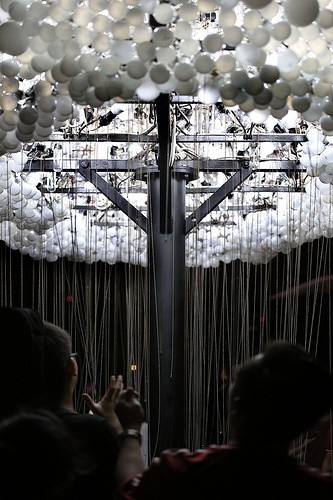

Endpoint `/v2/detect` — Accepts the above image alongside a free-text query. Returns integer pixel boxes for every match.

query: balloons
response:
[0,0,333,269]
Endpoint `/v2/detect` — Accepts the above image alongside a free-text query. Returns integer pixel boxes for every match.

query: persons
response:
[114,341,333,500]
[0,306,124,500]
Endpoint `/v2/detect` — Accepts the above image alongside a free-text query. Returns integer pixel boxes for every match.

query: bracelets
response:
[120,429,142,446]
